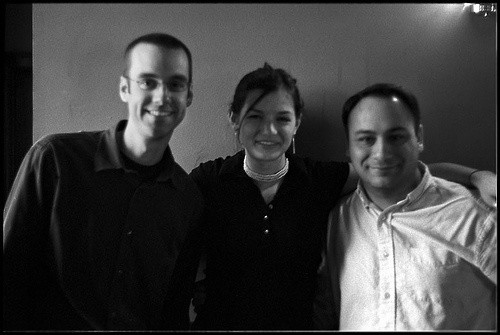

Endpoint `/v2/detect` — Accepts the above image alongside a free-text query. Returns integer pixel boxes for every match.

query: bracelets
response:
[469,169,482,187]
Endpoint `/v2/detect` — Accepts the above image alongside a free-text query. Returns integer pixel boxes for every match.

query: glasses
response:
[127,76,189,92]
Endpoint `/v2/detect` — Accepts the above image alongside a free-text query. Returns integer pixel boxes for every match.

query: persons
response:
[188,62,497,332]
[2,33,203,332]
[314,83,497,332]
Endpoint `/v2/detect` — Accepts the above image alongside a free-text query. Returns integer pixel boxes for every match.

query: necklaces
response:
[242,155,289,181]
